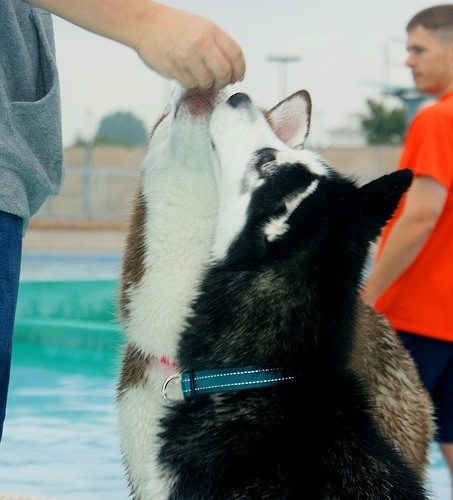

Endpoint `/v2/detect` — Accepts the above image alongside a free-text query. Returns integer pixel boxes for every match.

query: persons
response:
[358,4,453,478]
[0,0,244,445]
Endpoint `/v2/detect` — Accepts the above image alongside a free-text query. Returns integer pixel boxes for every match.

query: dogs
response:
[115,79,440,500]
[152,92,430,499]
